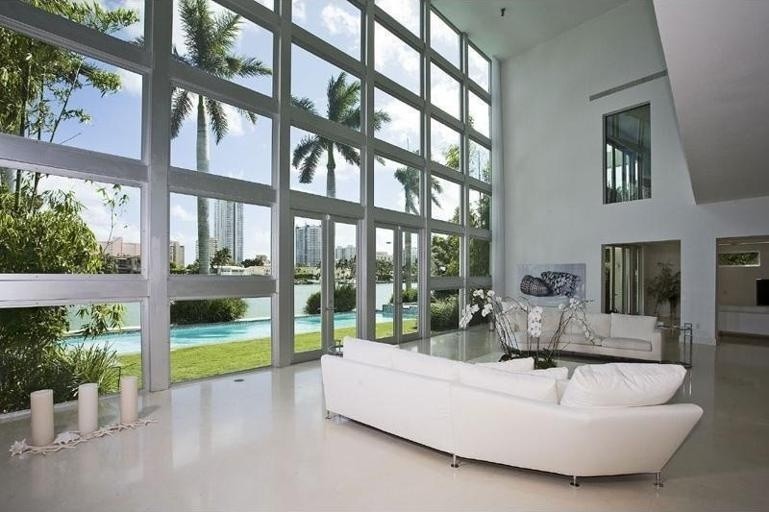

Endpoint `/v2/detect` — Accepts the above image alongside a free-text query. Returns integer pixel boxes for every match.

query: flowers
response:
[457,289,599,339]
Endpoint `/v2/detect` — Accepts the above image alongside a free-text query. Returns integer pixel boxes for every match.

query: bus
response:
[221,266,245,275]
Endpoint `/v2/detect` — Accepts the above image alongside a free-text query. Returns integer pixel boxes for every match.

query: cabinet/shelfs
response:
[717,306,769,340]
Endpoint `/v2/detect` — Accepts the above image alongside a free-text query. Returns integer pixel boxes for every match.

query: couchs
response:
[319,305,703,489]
